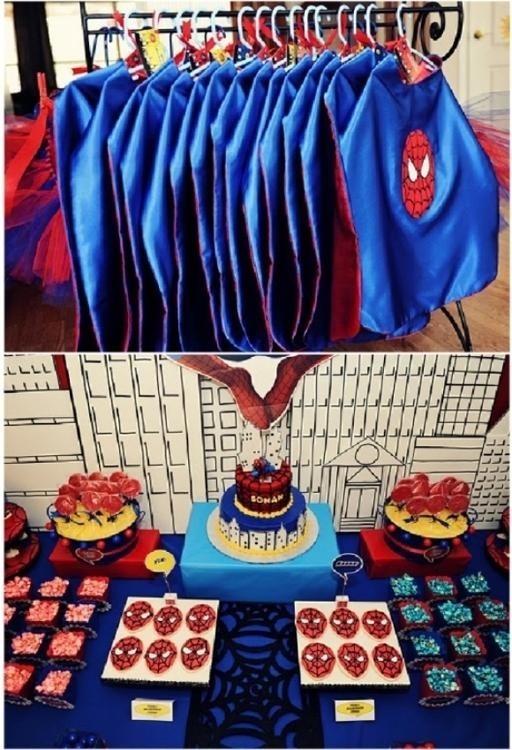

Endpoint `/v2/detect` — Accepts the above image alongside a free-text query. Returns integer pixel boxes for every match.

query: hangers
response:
[113,2,440,66]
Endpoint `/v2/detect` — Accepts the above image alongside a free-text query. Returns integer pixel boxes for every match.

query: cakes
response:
[5,502,31,566]
[384,473,471,539]
[217,455,308,552]
[47,471,142,542]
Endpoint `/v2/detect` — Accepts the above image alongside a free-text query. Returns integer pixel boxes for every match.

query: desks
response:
[6,521,509,747]
